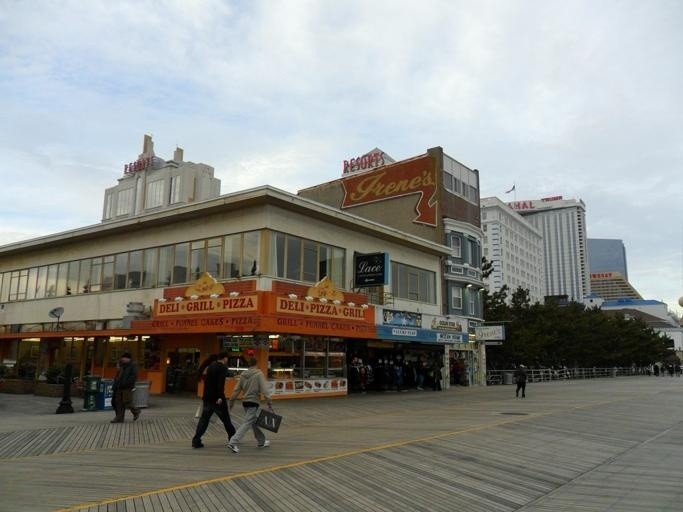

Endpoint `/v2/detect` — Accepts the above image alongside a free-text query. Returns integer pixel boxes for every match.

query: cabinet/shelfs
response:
[220,335,347,380]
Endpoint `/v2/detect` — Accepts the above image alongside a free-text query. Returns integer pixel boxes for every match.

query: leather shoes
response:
[133,415,138,420]
[191,443,203,448]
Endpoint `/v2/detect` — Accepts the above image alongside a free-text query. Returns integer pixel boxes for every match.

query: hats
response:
[520,365,524,368]
[246,356,256,366]
[218,351,230,357]
[121,353,131,359]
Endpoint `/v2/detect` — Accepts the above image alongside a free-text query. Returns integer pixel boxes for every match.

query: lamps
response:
[158,291,239,304]
[479,287,484,290]
[289,293,368,311]
[466,283,472,287]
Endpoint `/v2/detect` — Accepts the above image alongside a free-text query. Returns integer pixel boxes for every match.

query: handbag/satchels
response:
[512,375,517,384]
[255,408,282,433]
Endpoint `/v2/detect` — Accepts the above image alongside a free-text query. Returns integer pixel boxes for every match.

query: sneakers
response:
[227,444,239,453]
[258,440,270,448]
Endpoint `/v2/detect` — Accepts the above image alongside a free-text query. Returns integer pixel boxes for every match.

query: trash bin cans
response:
[504,373,514,385]
[82,375,151,411]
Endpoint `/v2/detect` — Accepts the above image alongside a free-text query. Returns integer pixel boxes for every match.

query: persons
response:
[110,353,140,423]
[192,351,236,448]
[225,356,272,452]
[395,358,463,392]
[195,353,217,418]
[513,364,527,397]
[110,359,122,416]
[645,361,682,377]
